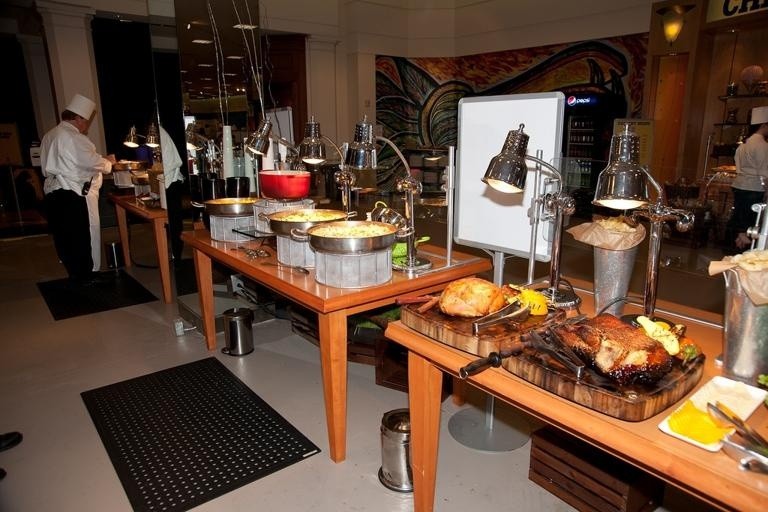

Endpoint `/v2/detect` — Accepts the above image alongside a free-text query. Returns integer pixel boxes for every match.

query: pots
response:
[257,209,416,254]
[108,162,150,186]
[190,198,263,216]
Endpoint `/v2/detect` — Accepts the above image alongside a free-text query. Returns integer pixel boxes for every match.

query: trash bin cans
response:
[664,179,701,209]
[104,240,125,269]
[221,307,255,356]
[320,160,355,203]
[378,408,413,493]
[668,200,711,247]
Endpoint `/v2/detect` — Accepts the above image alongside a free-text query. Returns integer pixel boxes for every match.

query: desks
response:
[113,190,184,300]
[383,275,768,512]
[179,224,490,465]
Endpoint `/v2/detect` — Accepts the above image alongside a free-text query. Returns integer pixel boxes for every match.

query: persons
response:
[720,105,768,256]
[0,430,23,482]
[39,92,118,289]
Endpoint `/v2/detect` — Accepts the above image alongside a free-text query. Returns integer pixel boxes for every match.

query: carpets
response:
[34,268,161,325]
[80,354,324,512]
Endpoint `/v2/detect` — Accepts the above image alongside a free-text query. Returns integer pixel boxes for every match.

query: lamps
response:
[484,121,581,311]
[183,120,244,198]
[594,118,695,318]
[123,122,167,211]
[342,114,432,271]
[247,116,357,222]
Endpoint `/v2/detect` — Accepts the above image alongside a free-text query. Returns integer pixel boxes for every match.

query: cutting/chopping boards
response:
[401,284,705,423]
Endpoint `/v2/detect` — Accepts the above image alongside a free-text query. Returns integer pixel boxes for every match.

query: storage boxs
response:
[284,303,452,401]
[523,427,662,512]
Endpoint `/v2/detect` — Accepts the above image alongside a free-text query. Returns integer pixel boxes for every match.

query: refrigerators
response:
[562,90,628,198]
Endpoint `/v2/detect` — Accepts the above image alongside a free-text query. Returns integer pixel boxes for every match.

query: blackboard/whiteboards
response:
[454,91,565,263]
[0,123,24,167]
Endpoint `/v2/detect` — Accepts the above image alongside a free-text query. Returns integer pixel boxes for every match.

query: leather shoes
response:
[0,430,23,452]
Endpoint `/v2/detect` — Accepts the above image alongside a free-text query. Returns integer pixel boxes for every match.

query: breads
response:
[555,312,673,388]
[438,277,506,319]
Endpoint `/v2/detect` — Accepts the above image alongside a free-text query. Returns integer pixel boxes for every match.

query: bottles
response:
[568,116,597,187]
[736,127,746,144]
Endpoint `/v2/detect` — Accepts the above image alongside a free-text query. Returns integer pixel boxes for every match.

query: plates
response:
[658,376,767,452]
[712,166,737,172]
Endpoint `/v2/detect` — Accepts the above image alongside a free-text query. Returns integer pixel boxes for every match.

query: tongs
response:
[706,400,767,455]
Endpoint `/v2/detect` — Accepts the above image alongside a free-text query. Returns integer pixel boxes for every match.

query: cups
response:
[592,246,637,316]
[726,81,767,125]
[190,172,249,198]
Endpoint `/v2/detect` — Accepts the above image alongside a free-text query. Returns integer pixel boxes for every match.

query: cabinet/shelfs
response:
[708,91,768,158]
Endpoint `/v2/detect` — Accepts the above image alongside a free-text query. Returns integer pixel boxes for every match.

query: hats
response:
[65,94,97,120]
[750,106,768,125]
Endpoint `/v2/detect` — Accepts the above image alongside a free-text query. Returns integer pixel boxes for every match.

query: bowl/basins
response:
[372,201,430,258]
[141,197,161,209]
[258,169,310,199]
[666,184,700,199]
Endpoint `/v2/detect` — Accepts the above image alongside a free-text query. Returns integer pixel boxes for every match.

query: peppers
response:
[676,337,703,366]
